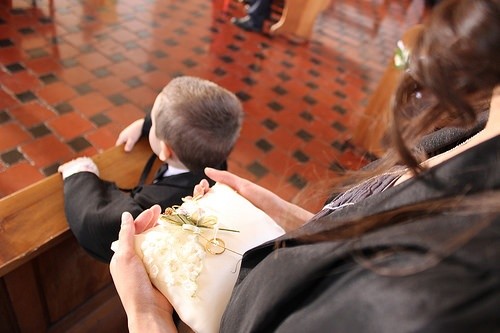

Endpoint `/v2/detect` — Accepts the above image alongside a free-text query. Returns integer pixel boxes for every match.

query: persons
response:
[232,0,272,33]
[109,0,500,333]
[58,75,243,261]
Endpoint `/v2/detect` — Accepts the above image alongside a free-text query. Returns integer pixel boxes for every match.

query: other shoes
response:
[231,14,263,31]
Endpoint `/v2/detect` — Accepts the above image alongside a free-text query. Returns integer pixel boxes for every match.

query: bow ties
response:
[152,163,168,180]
